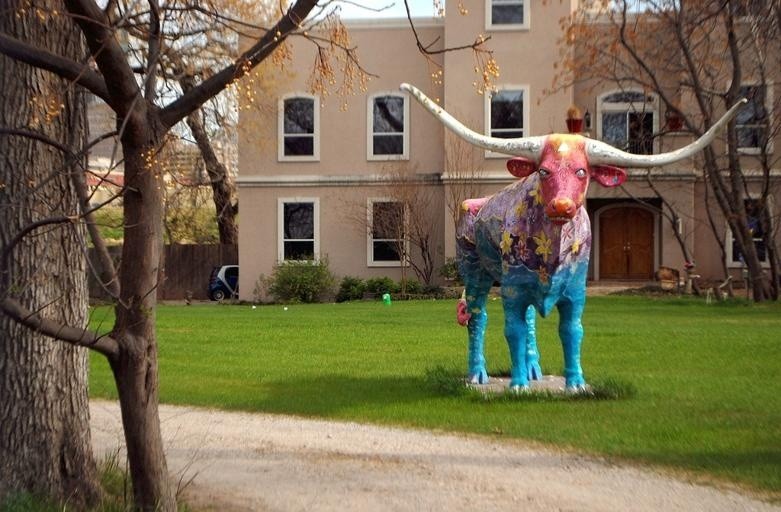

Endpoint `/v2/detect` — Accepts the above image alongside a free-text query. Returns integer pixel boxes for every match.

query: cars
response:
[208,264,238,302]
